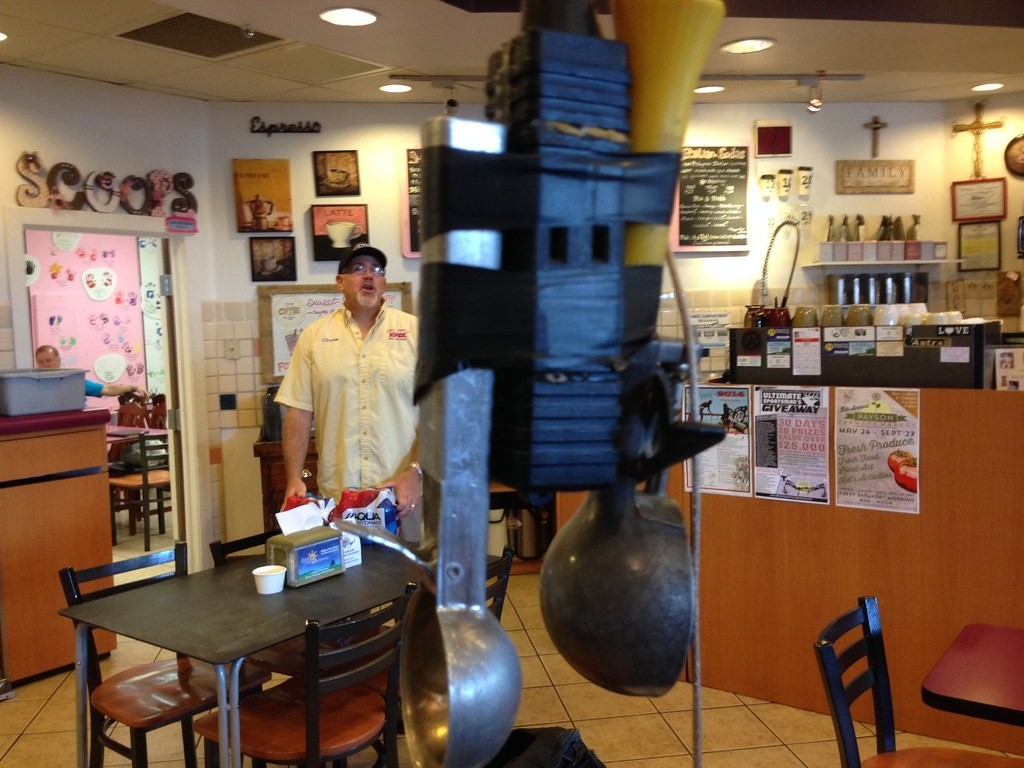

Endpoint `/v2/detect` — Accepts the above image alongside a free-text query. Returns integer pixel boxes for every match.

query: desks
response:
[922,624,1024,729]
[106,422,168,444]
[57,554,436,768]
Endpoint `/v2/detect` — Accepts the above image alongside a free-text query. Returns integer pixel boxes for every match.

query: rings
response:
[411,504,416,508]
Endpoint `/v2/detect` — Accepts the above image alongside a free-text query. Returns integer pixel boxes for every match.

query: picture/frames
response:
[248,236,298,282]
[952,176,1007,221]
[310,204,370,262]
[311,150,361,197]
[959,222,1002,270]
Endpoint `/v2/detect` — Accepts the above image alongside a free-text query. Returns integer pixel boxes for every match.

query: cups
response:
[790,302,963,325]
[258,240,277,271]
[253,564,287,594]
[326,222,363,248]
[328,167,348,183]
[762,307,791,328]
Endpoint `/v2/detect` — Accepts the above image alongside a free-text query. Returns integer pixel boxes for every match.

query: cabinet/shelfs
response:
[0,409,117,689]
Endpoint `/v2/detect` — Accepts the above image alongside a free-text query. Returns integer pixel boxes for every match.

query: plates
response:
[324,178,350,188]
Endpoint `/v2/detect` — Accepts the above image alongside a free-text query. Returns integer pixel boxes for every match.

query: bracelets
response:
[132,383,138,391]
[408,462,423,479]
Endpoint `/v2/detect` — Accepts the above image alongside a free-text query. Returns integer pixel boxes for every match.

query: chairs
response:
[108,391,173,551]
[814,595,1024,768]
[191,582,417,768]
[209,528,333,677]
[367,545,515,736]
[59,540,272,768]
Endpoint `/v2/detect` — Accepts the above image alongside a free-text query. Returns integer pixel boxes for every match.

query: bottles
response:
[744,304,766,327]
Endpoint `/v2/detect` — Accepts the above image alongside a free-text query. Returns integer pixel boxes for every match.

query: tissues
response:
[265,502,345,588]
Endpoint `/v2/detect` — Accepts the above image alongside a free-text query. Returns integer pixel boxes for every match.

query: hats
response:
[338,243,388,276]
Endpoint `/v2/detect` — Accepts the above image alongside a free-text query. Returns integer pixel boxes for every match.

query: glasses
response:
[342,264,386,277]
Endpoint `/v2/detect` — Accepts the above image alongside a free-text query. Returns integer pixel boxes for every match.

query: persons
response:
[36,345,151,409]
[722,404,733,431]
[700,400,714,421]
[273,242,424,735]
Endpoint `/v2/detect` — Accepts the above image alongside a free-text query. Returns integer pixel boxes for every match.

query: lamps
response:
[806,85,824,112]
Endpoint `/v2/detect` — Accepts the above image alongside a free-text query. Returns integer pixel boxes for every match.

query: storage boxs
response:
[820,240,948,262]
[0,368,92,417]
